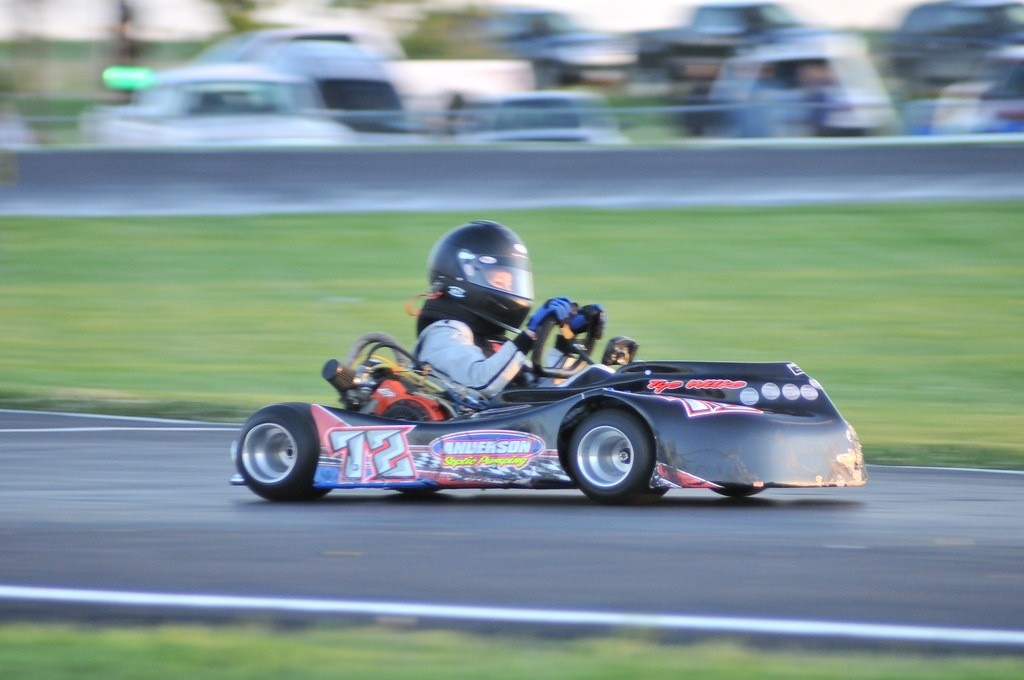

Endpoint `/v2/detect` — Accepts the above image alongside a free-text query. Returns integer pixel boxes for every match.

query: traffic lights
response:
[98,64,150,90]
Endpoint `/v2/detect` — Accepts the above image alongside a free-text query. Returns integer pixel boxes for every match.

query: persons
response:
[414,221,605,406]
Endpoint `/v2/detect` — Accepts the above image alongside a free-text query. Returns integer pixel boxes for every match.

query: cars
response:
[79,0,1022,145]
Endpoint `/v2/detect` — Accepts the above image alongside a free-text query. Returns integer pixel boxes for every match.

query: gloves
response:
[568,304,605,336]
[526,297,576,333]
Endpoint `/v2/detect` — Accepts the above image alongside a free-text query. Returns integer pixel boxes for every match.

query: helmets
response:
[428,220,535,335]
